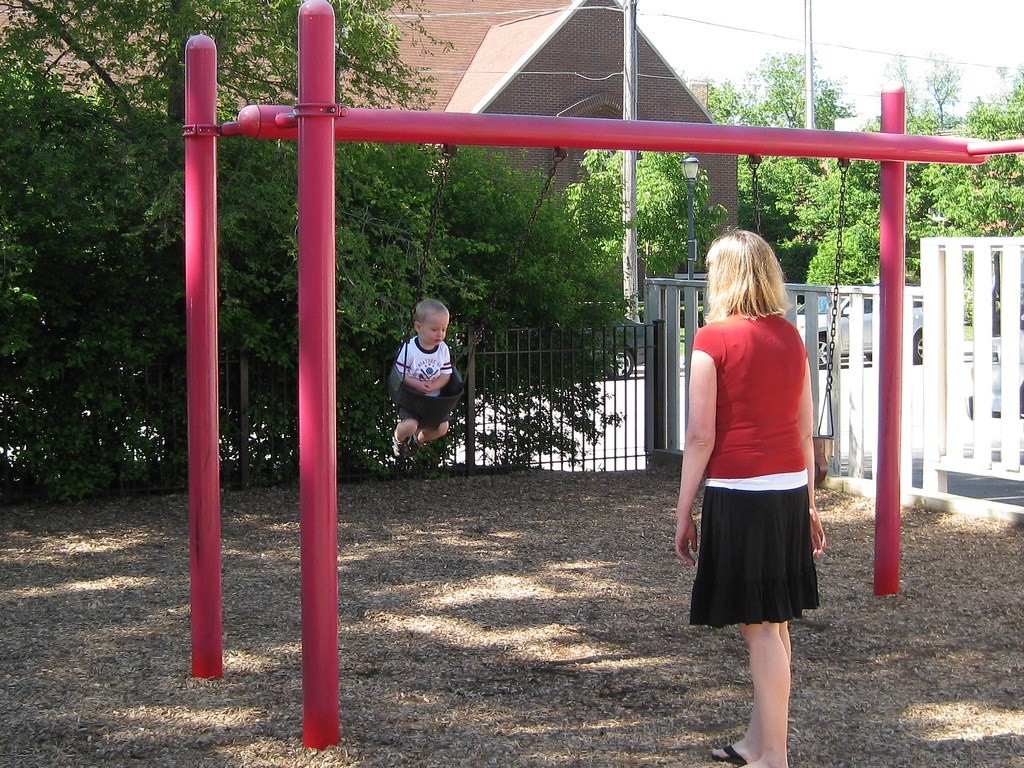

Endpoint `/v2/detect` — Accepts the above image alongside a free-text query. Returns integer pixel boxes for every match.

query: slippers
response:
[711,745,748,765]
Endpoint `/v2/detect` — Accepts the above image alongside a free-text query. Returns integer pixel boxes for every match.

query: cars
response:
[796,284,922,365]
[574,312,646,382]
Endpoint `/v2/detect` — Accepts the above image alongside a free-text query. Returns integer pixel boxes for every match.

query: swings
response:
[751,163,848,487]
[388,150,566,429]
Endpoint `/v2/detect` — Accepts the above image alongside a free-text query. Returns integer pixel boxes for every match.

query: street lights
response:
[678,154,701,280]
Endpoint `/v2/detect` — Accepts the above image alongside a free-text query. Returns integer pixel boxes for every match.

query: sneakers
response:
[392,431,407,459]
[407,427,425,456]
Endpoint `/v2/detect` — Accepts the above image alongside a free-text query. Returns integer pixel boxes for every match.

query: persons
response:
[392,299,452,458]
[675,230,827,768]
